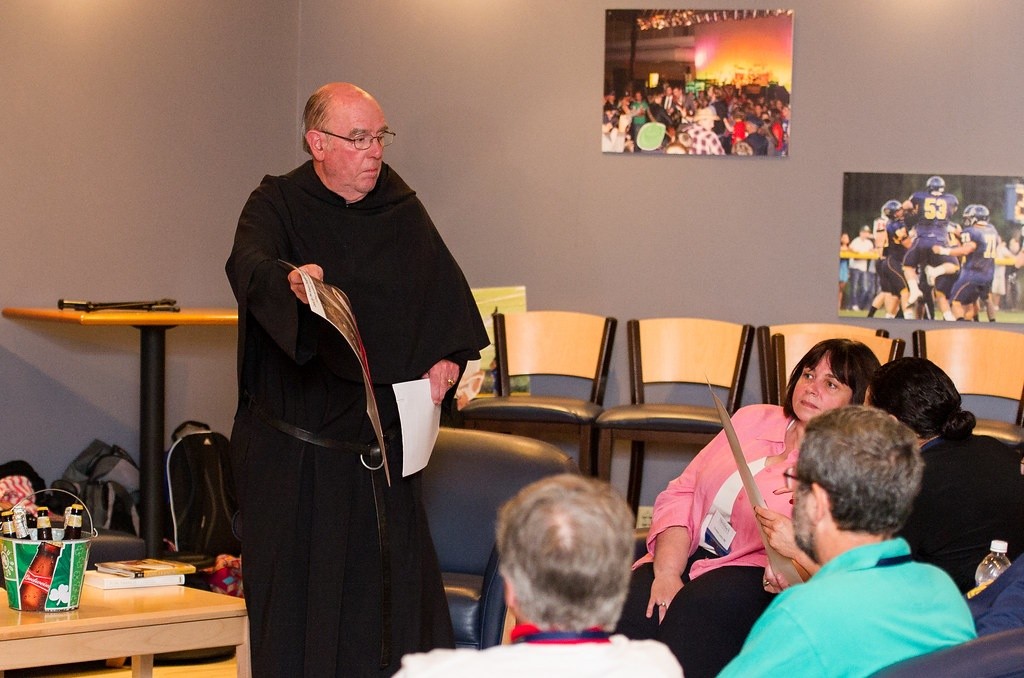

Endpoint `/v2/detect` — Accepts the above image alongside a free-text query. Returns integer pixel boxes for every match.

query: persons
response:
[601,80,791,157]
[839,176,1024,323]
[391,338,1024,678]
[225,83,491,677]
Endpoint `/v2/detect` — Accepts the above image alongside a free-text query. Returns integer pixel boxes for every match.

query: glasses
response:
[783,467,842,496]
[317,131,396,150]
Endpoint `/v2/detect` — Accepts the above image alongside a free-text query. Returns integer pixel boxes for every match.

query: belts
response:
[239,398,401,669]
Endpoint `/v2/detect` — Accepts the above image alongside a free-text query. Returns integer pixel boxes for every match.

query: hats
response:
[859,224,871,232]
[741,112,763,127]
[636,122,666,150]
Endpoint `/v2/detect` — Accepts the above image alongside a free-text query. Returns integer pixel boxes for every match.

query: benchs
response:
[0,521,147,667]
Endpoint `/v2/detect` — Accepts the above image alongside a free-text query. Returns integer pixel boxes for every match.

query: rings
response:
[449,379,455,386]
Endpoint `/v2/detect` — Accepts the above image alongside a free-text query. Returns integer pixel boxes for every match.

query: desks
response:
[0,570,253,678]
[0,306,242,668]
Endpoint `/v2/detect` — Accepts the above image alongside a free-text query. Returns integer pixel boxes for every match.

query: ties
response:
[666,99,670,110]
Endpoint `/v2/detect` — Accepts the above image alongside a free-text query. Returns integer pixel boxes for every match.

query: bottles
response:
[974,539,1012,588]
[19,541,65,611]
[1,502,84,540]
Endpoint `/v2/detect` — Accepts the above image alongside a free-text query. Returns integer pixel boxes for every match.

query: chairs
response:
[415,313,1024,678]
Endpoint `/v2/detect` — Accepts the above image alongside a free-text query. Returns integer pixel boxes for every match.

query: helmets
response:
[944,194,959,206]
[926,176,945,189]
[883,200,900,216]
[963,204,991,218]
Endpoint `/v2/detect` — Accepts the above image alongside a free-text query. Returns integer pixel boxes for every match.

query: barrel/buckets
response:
[0,489,98,612]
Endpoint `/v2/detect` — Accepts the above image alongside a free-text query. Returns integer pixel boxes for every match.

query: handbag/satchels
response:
[51,440,141,515]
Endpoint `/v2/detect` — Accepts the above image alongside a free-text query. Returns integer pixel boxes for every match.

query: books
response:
[84,558,197,589]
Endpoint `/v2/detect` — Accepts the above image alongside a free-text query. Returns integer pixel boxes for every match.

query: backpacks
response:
[76,480,140,538]
[161,419,238,560]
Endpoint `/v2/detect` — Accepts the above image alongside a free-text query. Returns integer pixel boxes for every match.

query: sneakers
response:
[905,291,923,308]
[924,265,935,288]
